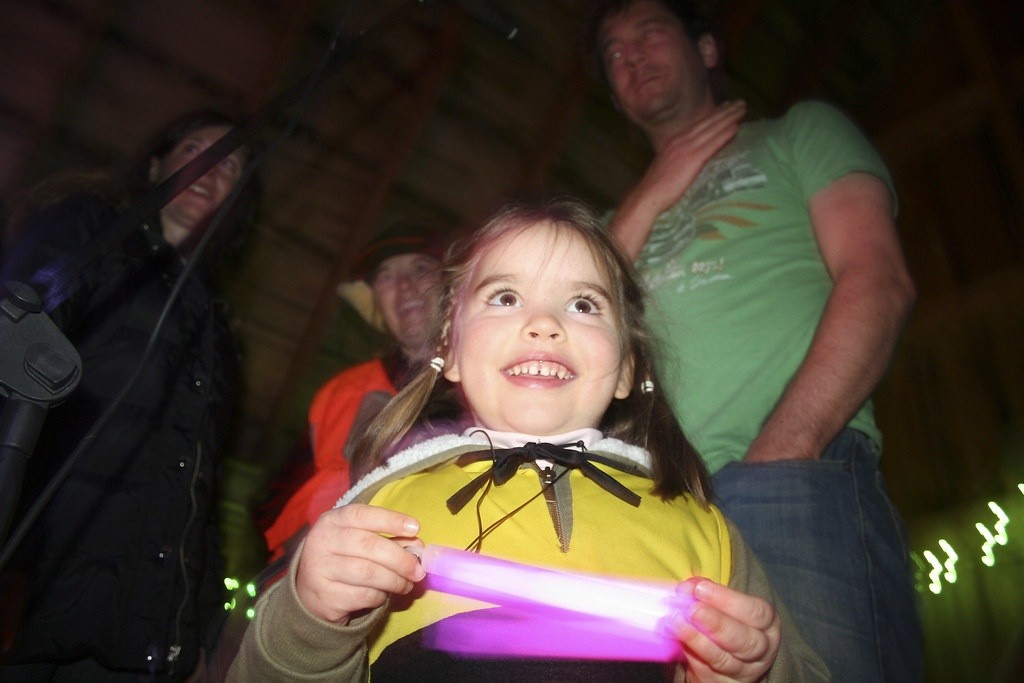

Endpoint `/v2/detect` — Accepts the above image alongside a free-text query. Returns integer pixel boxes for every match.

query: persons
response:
[253,224,475,596]
[0,109,266,683]
[592,0,935,682]
[217,199,833,683]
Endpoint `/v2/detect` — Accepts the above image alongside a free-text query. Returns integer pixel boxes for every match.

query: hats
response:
[336,221,453,336]
